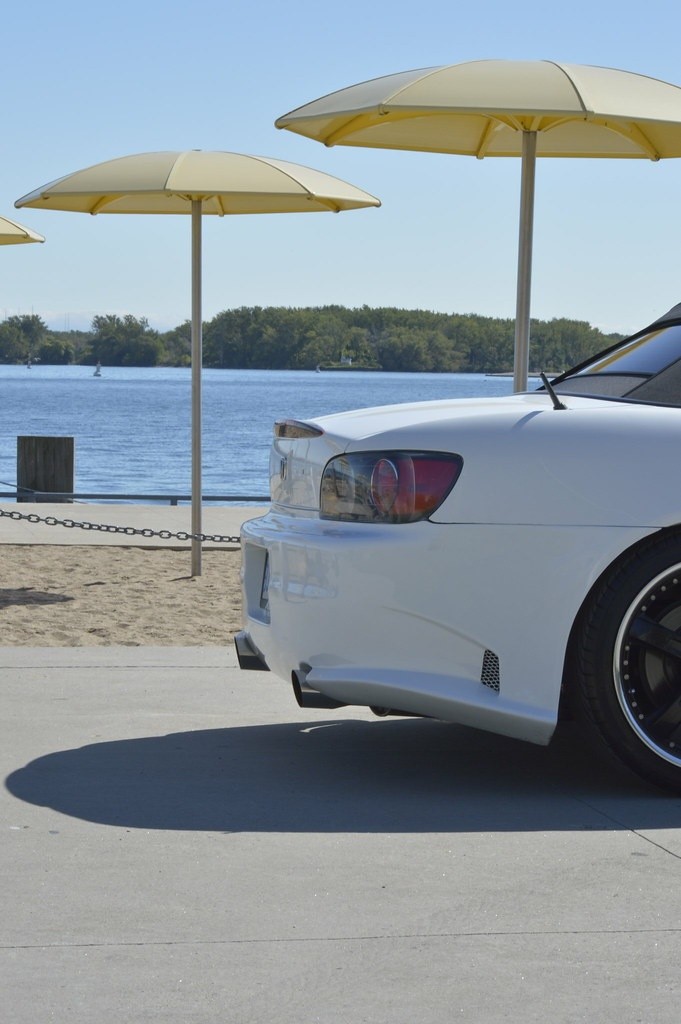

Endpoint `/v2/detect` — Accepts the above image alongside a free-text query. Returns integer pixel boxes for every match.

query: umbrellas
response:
[272,58,681,395]
[14,147,385,579]
[0,214,46,247]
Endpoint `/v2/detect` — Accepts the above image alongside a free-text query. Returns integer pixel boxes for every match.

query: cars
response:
[225,303,681,798]
